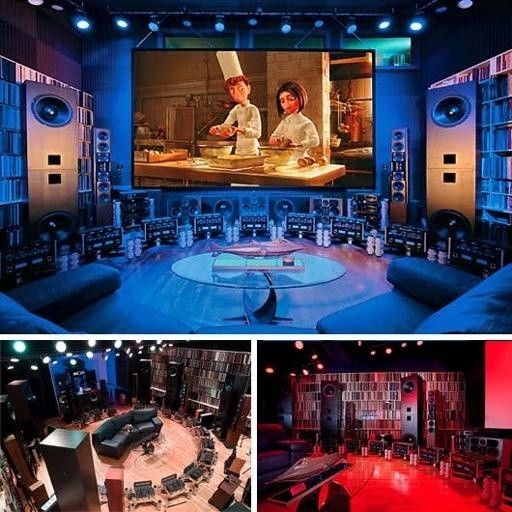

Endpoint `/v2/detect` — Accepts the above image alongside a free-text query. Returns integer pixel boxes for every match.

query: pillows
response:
[257,429,281,448]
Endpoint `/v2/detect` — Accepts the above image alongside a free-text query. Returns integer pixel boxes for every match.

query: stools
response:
[417,445,444,465]
[392,441,414,459]
[499,466,512,505]
[450,449,498,480]
[368,440,388,454]
[344,439,361,451]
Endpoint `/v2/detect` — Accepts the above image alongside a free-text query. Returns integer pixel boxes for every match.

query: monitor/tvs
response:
[132,47,376,192]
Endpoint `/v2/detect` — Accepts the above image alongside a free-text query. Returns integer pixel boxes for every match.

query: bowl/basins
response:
[258,145,295,164]
[197,143,232,162]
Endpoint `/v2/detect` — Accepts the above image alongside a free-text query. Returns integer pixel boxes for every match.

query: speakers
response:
[137,358,151,406]
[38,429,99,511]
[321,381,341,452]
[165,361,182,412]
[428,391,437,444]
[213,373,250,439]
[426,80,477,247]
[9,380,44,438]
[0,394,36,488]
[390,127,409,222]
[401,375,422,445]
[345,401,355,433]
[94,127,111,227]
[25,80,79,246]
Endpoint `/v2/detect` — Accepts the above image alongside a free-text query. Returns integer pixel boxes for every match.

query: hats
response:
[216,49,243,82]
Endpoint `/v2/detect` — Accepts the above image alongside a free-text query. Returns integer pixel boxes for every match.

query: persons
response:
[268,81,320,155]
[208,50,261,156]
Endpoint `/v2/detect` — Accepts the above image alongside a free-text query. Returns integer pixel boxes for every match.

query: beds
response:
[172,251,347,325]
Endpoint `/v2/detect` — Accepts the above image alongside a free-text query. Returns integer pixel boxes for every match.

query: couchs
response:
[257,424,313,500]
[92,407,163,460]
[0,257,512,334]
